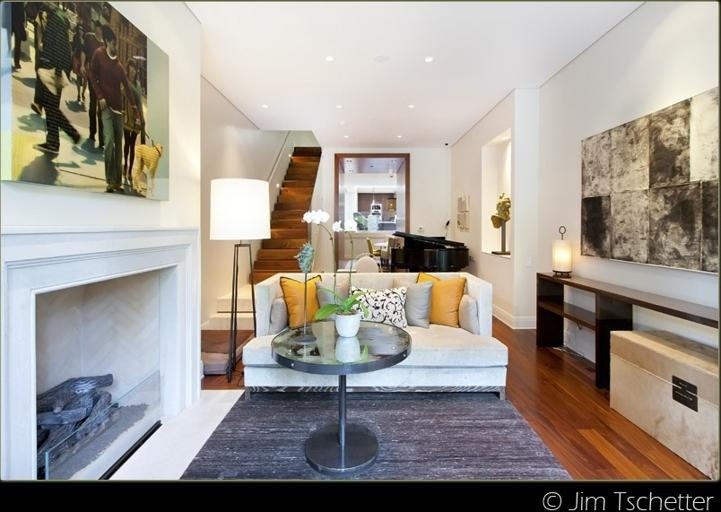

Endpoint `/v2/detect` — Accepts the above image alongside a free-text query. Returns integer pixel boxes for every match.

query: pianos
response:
[392,232,469,272]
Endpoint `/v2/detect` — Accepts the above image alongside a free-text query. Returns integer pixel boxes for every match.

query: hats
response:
[37,66,71,96]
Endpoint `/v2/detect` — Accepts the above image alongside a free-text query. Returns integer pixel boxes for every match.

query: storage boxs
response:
[605,322,721,480]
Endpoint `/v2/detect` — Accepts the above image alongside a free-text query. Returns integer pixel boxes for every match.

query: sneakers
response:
[34,142,60,155]
[30,102,43,117]
[99,146,104,151]
[15,65,22,72]
[106,183,126,194]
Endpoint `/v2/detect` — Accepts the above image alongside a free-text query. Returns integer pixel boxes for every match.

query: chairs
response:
[380,237,404,269]
[366,235,380,259]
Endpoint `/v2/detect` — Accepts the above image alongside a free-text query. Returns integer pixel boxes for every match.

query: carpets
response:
[180,388,578,479]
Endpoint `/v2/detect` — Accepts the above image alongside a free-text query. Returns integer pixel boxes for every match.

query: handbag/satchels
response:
[24,3,41,19]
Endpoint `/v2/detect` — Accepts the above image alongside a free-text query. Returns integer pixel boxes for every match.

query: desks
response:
[269,317,416,472]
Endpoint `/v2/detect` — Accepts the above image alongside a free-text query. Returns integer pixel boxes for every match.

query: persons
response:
[10,1,145,191]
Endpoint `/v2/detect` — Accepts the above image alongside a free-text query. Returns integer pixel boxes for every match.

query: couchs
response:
[240,265,512,402]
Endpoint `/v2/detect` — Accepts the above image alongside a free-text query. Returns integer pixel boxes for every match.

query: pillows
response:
[279,274,324,329]
[459,291,480,336]
[399,281,433,331]
[417,270,467,330]
[346,285,410,330]
[316,277,352,309]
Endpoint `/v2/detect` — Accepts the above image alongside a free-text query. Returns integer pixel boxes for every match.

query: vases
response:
[331,313,363,340]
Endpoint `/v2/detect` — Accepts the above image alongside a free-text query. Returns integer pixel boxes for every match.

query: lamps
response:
[551,224,578,282]
[207,175,277,385]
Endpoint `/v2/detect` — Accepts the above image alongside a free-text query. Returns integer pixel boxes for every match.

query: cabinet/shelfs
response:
[531,269,721,414]
[336,229,397,261]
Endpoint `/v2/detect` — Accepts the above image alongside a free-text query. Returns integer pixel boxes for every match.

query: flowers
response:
[299,205,373,320]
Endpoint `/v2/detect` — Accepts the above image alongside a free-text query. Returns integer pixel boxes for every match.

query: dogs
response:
[131,144,163,193]
